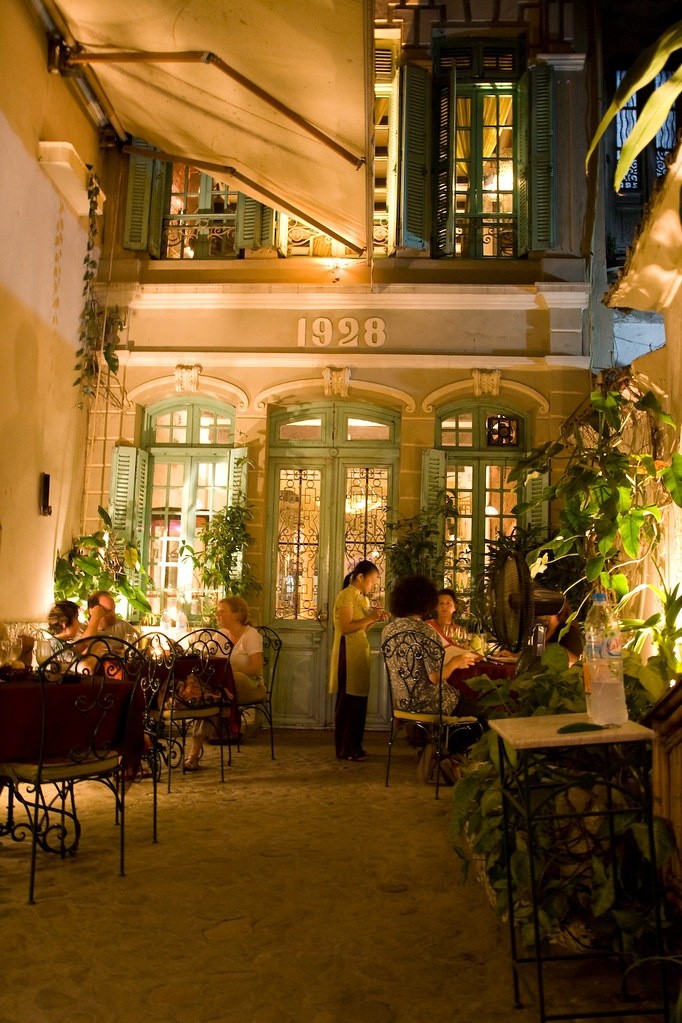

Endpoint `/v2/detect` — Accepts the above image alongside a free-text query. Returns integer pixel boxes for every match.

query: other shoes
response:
[184,756,199,770]
[440,756,462,786]
[336,751,368,761]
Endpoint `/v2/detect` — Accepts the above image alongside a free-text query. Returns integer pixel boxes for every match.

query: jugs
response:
[530,623,547,658]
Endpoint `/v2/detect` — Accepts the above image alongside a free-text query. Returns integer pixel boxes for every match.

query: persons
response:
[495,585,586,668]
[183,596,267,770]
[328,560,390,761]
[48,591,167,780]
[381,575,476,786]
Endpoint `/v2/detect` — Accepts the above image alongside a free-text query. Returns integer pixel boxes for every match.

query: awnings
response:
[37,0,374,259]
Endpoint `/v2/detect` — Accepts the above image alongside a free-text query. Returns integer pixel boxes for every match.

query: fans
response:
[489,547,565,657]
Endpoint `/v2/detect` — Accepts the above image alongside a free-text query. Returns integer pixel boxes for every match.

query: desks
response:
[488,712,664,1023]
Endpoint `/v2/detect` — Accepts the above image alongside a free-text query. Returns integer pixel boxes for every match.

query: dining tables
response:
[446,661,520,757]
[0,671,146,854]
[97,656,237,783]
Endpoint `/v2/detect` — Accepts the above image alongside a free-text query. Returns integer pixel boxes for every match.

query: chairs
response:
[0,634,148,905]
[143,629,234,794]
[58,632,176,860]
[225,625,282,766]
[380,629,479,799]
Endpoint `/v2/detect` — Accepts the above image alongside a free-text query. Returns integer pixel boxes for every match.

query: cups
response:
[443,624,487,651]
[0,638,74,674]
[124,631,153,658]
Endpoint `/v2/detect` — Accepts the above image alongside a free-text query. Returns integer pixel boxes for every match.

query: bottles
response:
[176,610,189,650]
[581,593,629,725]
[159,608,171,651]
